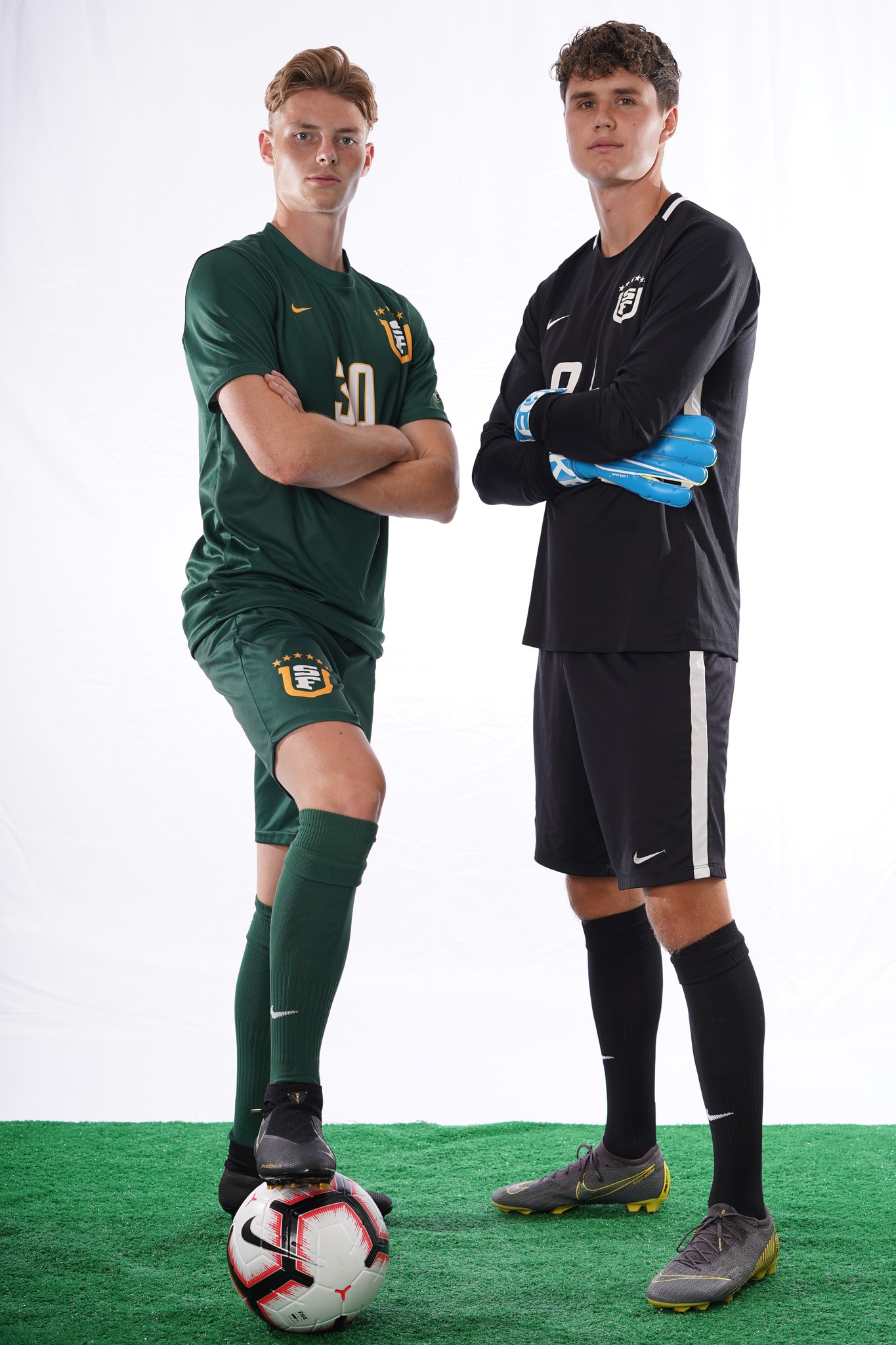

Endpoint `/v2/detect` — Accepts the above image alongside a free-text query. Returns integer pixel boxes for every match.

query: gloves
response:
[513,387,570,443]
[549,414,719,509]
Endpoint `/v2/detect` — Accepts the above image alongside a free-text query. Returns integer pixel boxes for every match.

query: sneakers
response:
[217,1143,393,1219]
[491,1139,672,1215]
[254,1081,338,1192]
[645,1202,780,1313]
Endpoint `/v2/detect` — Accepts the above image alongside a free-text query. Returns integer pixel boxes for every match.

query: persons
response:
[180,44,461,1215]
[472,20,784,1316]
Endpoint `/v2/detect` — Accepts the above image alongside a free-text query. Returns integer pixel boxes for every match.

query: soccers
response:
[226,1172,390,1335]
